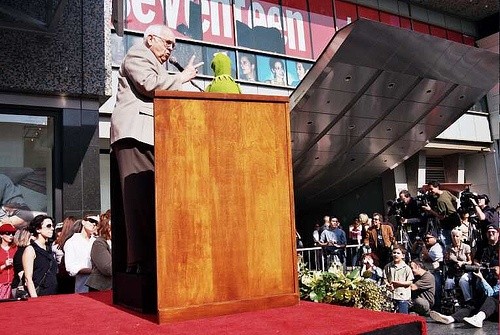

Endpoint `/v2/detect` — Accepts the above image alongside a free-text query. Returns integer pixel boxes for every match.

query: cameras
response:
[463,262,495,273]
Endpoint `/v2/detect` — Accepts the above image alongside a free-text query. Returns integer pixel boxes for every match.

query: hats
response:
[55,223,63,230]
[0,224,16,233]
[487,224,500,232]
[428,232,438,238]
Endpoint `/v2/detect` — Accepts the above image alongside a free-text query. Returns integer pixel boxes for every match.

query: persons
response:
[297,182,500,329]
[109,22,207,308]
[237,52,257,82]
[265,57,287,85]
[290,63,312,86]
[0,207,112,304]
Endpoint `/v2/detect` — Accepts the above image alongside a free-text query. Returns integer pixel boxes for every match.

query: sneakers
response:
[463,314,483,328]
[430,311,454,324]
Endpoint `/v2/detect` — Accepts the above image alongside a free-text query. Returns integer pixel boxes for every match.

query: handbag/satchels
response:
[12,271,40,299]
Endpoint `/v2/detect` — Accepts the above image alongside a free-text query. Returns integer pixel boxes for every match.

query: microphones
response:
[168,56,184,72]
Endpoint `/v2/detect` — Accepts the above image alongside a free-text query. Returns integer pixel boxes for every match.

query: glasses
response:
[373,218,378,220]
[5,233,15,236]
[47,224,54,228]
[153,34,175,49]
[425,237,433,239]
[89,218,98,226]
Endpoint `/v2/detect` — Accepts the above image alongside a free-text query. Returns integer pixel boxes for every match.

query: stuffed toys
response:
[205,52,242,94]
